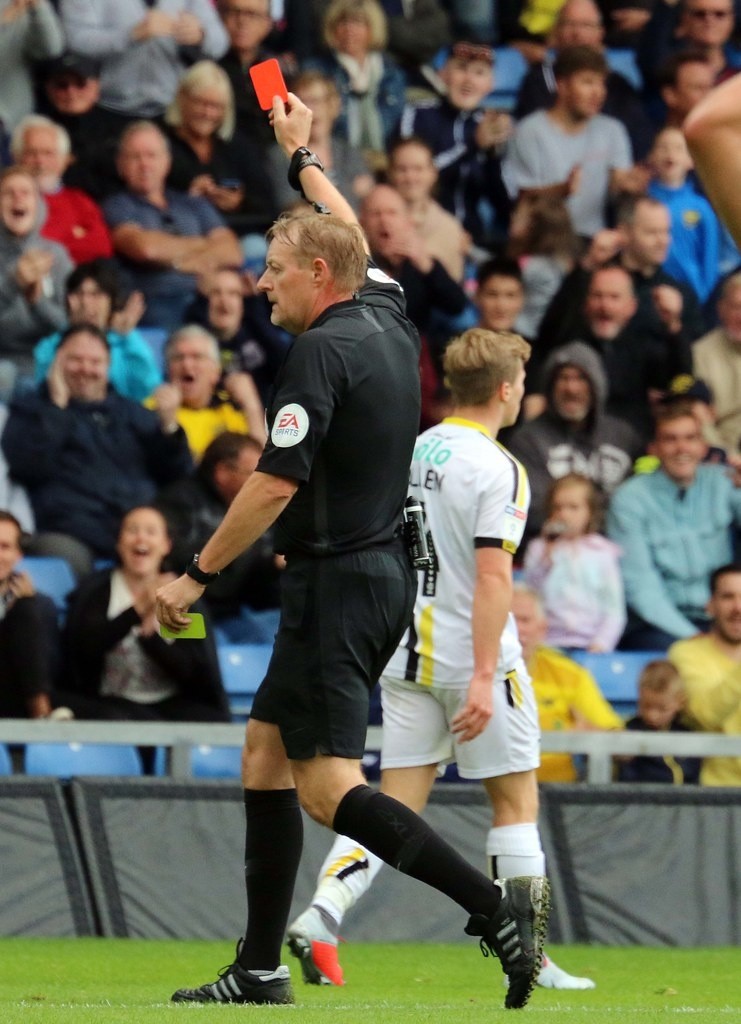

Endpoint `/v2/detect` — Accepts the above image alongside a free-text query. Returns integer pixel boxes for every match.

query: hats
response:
[646,373,714,406]
[50,47,99,76]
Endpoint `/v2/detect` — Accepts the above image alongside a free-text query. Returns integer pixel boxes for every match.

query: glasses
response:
[51,80,90,90]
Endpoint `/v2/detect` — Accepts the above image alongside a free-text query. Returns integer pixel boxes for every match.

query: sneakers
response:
[466,877,551,1011]
[287,904,342,988]
[504,957,596,992]
[171,938,296,1005]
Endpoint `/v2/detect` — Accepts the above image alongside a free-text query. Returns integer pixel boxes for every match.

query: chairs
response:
[13,554,665,779]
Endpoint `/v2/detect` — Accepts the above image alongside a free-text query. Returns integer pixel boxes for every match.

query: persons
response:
[287,323,597,990]
[152,90,553,1008]
[0,0,741,791]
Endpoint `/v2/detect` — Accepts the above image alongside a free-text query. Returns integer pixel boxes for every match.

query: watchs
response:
[289,154,325,174]
[183,554,224,584]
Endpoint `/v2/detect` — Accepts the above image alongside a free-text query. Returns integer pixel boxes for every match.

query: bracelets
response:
[288,146,312,191]
